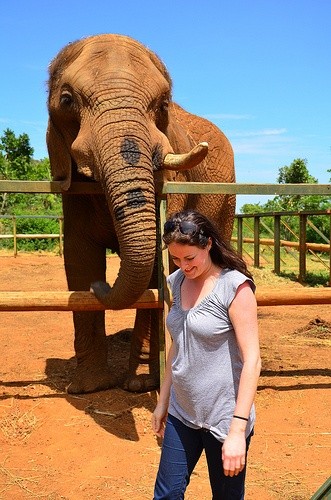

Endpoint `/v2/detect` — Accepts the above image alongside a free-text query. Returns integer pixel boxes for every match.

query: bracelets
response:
[232,415,249,422]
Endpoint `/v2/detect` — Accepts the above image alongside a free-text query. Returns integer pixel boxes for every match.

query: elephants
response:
[44,33,237,394]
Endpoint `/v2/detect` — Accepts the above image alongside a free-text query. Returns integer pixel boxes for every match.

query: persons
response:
[151,209,262,500]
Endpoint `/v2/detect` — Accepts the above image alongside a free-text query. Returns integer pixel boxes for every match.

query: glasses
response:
[162,219,211,239]
